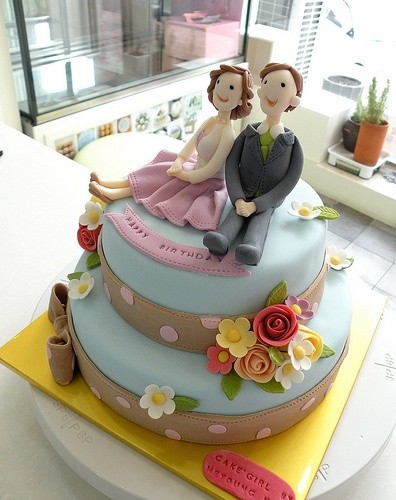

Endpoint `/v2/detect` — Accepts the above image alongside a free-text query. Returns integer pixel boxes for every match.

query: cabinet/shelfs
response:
[239,86,396,230]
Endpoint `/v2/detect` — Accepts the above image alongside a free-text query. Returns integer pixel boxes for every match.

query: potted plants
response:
[354,77,390,167]
[342,96,368,153]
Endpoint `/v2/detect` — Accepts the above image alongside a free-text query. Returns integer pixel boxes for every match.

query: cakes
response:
[64,62,355,446]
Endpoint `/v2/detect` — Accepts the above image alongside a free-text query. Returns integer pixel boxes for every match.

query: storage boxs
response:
[165,17,239,61]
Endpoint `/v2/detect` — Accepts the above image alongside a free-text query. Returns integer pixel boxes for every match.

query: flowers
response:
[77,195,108,269]
[206,280,336,401]
[67,272,95,300]
[139,384,200,419]
[298,206,340,220]
[328,253,354,270]
